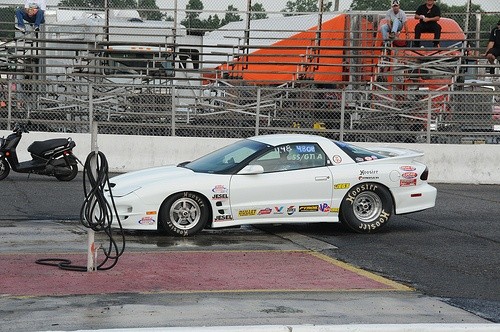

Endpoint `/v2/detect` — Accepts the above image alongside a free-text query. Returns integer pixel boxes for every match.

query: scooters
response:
[0,119,85,182]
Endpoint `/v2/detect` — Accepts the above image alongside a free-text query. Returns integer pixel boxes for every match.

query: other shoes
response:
[389,32,395,38]
[490,67,495,75]
[433,43,437,47]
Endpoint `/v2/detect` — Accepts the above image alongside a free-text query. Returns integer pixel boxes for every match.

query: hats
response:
[391,0,399,6]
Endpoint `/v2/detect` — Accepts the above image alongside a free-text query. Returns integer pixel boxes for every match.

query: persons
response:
[14,4,45,33]
[414,0,442,48]
[484,19,500,74]
[380,0,409,46]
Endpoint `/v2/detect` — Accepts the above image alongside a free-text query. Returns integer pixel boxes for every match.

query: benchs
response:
[0,23,500,144]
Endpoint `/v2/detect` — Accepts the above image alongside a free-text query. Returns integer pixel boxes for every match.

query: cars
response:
[82,133,439,239]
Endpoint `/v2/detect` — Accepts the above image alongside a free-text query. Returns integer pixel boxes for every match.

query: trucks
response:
[10,10,186,126]
[200,10,475,141]
